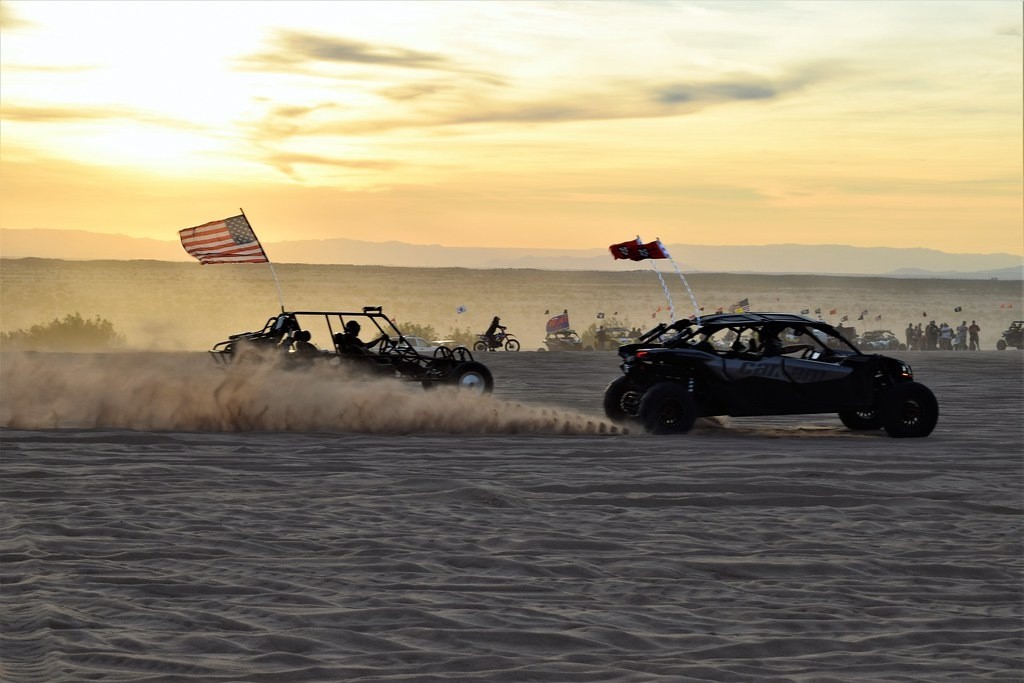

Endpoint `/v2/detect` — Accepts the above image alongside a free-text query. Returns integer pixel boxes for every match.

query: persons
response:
[342,320,423,376]
[484,314,507,353]
[904,320,981,351]
[835,323,845,349]
[723,329,737,342]
[756,326,816,359]
[595,325,643,351]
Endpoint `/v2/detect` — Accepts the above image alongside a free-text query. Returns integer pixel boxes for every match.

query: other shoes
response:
[490,349,495,352]
[409,366,429,377]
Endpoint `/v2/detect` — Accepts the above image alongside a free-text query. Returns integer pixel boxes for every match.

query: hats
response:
[599,326,604,329]
[494,316,501,320]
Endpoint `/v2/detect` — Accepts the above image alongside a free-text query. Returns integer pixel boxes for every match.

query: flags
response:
[176,214,269,267]
[546,313,570,333]
[727,297,751,315]
[608,236,672,264]
[954,306,963,313]
[799,306,883,323]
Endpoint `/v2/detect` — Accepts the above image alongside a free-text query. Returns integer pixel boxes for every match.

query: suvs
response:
[385,336,446,353]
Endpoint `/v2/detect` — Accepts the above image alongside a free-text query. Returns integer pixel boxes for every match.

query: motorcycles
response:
[595,328,642,350]
[857,330,900,350]
[473,328,520,351]
[997,321,1024,350]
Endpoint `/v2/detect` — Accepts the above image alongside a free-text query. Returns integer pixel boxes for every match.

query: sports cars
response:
[538,330,593,351]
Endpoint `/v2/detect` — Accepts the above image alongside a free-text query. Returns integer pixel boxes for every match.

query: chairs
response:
[731,338,757,351]
[293,330,318,350]
[333,333,364,354]
